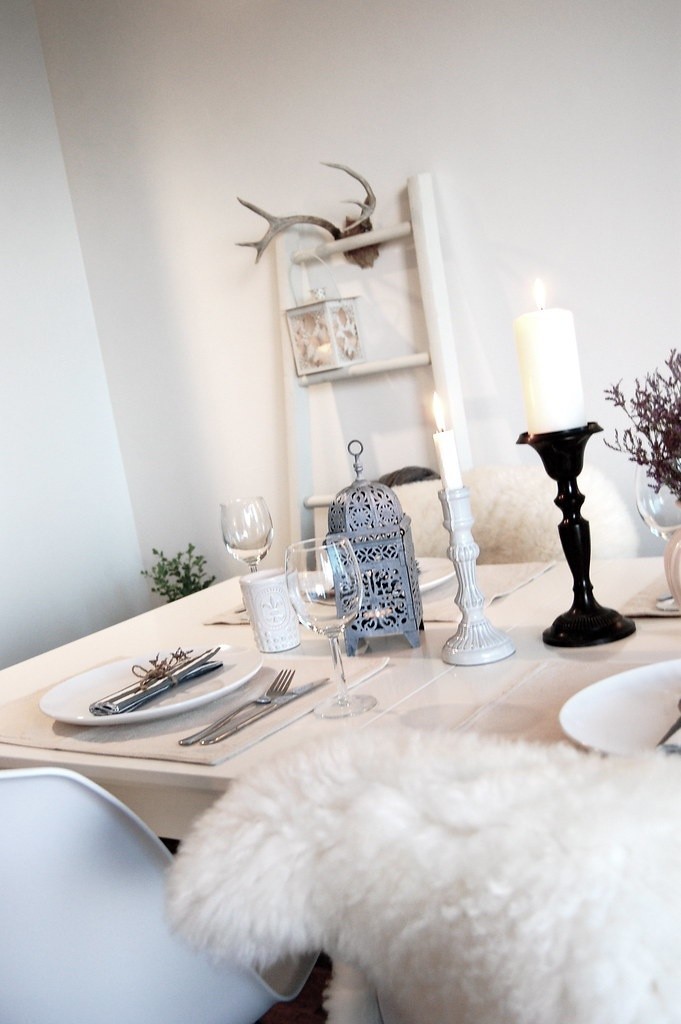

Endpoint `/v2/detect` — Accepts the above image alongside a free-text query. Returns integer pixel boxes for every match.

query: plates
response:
[40,644,265,725]
[559,659,681,757]
[416,555,455,593]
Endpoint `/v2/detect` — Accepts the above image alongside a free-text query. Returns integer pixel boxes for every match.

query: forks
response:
[179,670,296,745]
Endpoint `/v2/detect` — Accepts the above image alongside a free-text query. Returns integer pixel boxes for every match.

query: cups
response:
[240,568,300,652]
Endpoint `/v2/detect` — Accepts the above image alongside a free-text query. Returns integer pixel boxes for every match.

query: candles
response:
[513,276,588,436]
[431,392,463,492]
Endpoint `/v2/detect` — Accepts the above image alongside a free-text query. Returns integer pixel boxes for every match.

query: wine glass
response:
[635,457,681,610]
[219,496,273,619]
[284,536,377,719]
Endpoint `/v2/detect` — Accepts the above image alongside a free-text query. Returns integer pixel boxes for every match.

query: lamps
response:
[286,296,368,376]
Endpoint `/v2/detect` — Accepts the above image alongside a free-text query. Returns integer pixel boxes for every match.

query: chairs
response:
[0,768,324,1023]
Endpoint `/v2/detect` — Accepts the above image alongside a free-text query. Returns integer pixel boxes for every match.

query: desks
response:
[0,560,680,844]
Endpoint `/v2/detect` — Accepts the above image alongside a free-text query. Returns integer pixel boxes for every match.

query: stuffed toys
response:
[165,713,681,1024]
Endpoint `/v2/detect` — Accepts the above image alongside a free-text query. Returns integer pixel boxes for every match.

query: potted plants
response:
[602,349,681,613]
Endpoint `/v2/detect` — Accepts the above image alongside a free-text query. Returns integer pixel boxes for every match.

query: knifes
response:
[89,647,223,715]
[199,677,330,745]
